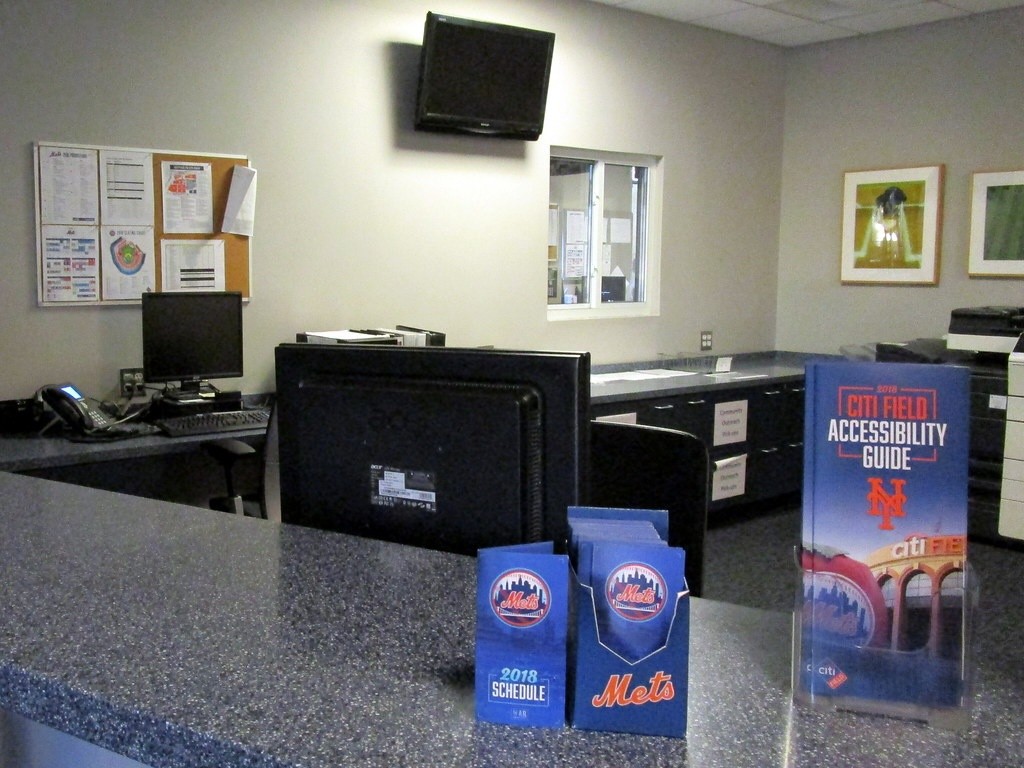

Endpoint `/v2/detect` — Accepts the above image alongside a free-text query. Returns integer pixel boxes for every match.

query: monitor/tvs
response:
[140,291,244,401]
[416,11,555,139]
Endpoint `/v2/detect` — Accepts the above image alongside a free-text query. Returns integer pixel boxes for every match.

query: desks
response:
[0,350,1024,768]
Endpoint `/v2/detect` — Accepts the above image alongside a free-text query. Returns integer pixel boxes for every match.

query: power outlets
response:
[118,368,146,396]
[700,329,712,351]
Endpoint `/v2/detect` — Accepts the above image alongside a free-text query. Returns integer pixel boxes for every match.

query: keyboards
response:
[154,410,270,438]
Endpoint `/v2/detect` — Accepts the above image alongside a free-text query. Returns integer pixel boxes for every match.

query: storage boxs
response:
[567,563,692,739]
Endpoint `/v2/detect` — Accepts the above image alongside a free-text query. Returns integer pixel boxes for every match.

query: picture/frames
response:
[836,163,945,286]
[966,167,1024,280]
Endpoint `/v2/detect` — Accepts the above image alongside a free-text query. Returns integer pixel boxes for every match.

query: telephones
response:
[40,382,113,437]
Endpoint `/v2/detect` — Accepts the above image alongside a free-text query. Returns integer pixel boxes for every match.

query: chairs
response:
[589,419,716,600]
[204,396,282,523]
